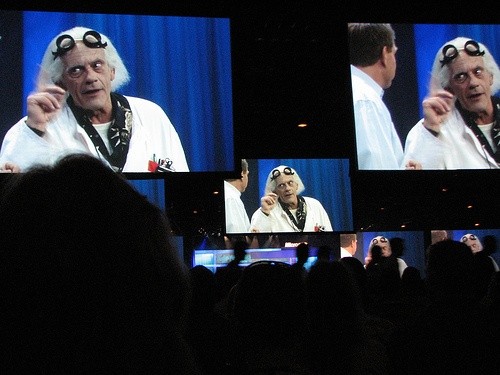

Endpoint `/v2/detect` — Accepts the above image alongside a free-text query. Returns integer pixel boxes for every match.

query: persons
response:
[347,23,500,170]
[190,235,500,375]
[223,158,333,234]
[0,155,196,375]
[0,24,192,172]
